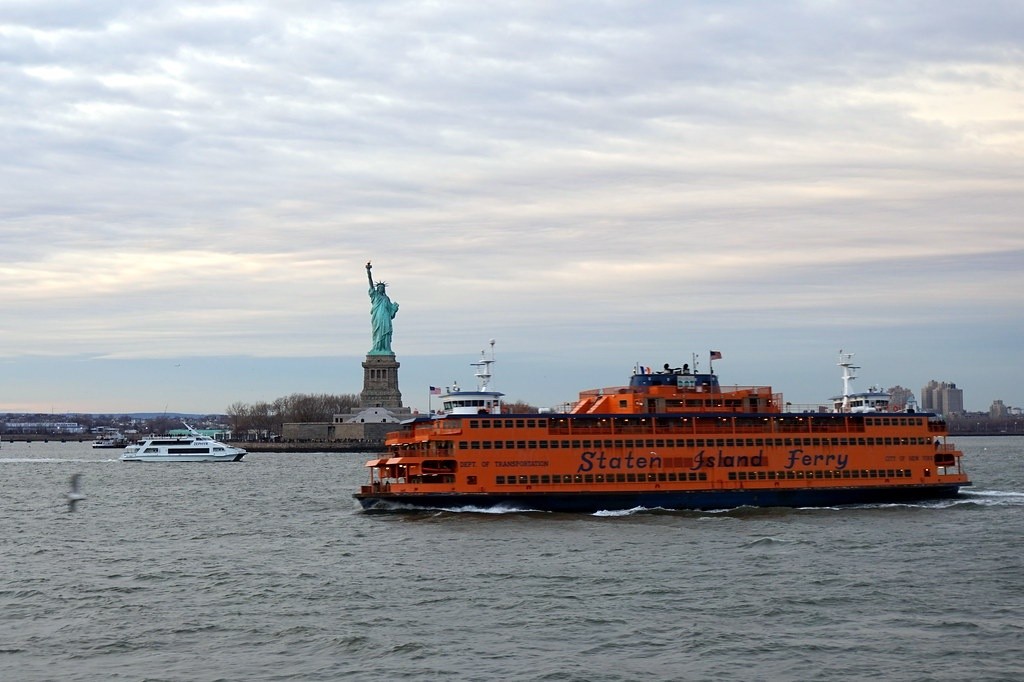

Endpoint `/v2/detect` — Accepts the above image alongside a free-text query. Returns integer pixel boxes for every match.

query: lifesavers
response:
[894,406,898,412]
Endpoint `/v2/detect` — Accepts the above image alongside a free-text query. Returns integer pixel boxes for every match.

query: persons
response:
[928,420,946,431]
[935,440,940,448]
[366,262,398,351]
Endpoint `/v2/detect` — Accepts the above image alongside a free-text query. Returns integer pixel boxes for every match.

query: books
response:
[391,302,399,315]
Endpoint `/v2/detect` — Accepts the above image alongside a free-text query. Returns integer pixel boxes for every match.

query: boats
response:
[119,434,248,462]
[351,337,972,512]
[90,438,127,449]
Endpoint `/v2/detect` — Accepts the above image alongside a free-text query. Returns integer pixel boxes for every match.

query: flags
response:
[430,387,441,394]
[711,351,721,360]
